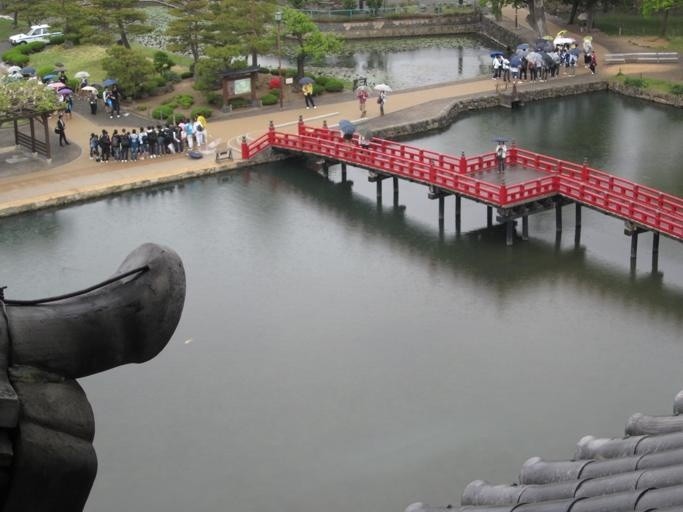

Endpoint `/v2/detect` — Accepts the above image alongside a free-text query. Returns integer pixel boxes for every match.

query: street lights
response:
[274,11,283,107]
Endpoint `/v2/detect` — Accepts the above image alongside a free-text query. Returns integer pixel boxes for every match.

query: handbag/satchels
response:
[55,128,63,134]
[376,98,381,104]
[495,148,502,160]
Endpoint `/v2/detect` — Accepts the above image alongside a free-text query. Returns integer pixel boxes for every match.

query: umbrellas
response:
[357,124,372,140]
[492,134,512,144]
[355,85,372,93]
[373,84,391,93]
[298,76,314,85]
[556,30,568,36]
[338,119,355,136]
[7,65,121,119]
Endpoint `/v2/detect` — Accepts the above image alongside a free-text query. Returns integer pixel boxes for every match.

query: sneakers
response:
[117,115,120,118]
[491,71,561,86]
[110,116,113,119]
[88,146,205,164]
[313,106,317,110]
[306,107,310,110]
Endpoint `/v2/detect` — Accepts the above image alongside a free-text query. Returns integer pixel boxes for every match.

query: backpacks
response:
[91,124,205,147]
[344,135,352,140]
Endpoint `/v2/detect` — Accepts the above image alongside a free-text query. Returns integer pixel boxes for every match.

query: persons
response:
[343,132,353,151]
[378,90,386,116]
[576,10,589,34]
[301,81,316,109]
[88,112,208,163]
[495,141,506,173]
[490,32,596,84]
[357,133,369,156]
[358,90,367,112]
[55,113,69,148]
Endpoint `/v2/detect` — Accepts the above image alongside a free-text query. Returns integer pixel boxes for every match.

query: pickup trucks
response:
[8,23,64,46]
